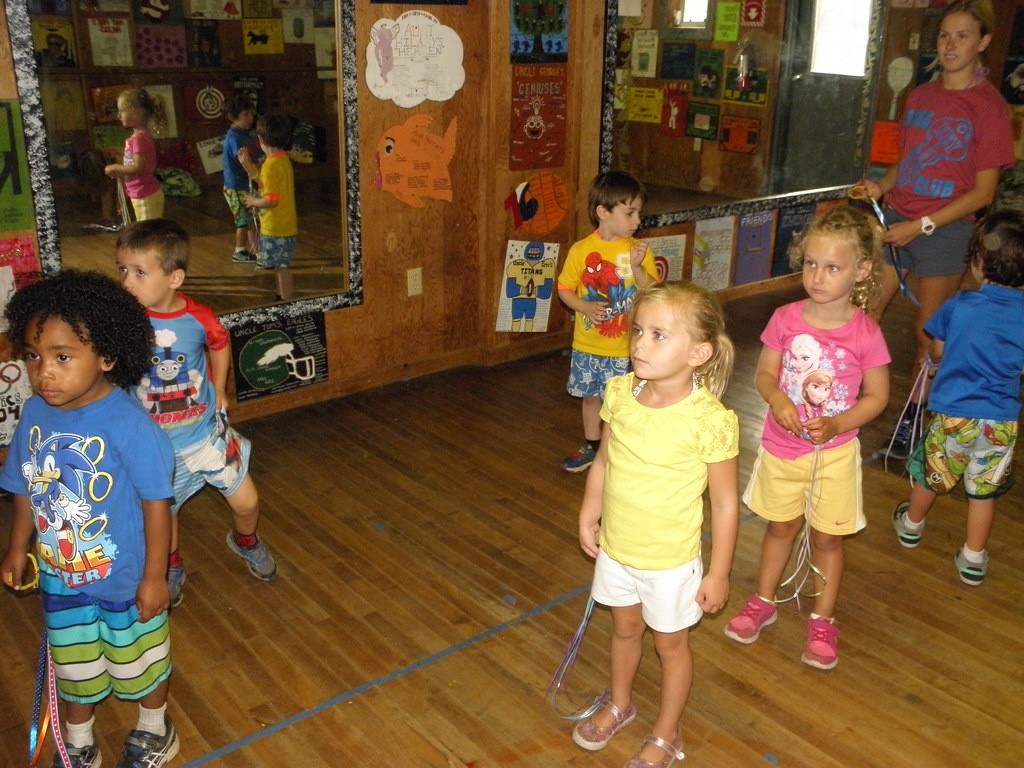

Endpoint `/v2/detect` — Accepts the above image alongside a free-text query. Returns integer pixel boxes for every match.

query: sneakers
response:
[724,595,779,644]
[953,543,990,586]
[878,416,929,461]
[559,443,601,473]
[892,498,928,548]
[165,564,188,608]
[800,616,842,669]
[49,742,103,768]
[113,713,181,768]
[226,527,278,581]
[232,249,259,263]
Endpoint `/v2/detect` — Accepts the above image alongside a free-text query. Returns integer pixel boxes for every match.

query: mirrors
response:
[4,0,363,331]
[598,0,894,232]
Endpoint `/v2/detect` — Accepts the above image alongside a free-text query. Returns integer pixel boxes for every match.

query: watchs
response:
[920,215,935,235]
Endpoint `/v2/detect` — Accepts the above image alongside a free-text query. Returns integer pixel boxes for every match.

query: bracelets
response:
[926,353,939,368]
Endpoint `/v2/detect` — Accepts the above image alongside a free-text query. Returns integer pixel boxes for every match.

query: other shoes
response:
[622,724,687,768]
[573,694,637,750]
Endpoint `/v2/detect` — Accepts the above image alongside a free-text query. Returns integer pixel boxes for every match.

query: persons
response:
[560,171,659,473]
[725,206,892,670]
[106,88,168,222]
[894,209,1024,585]
[566,281,739,757]
[116,218,279,608]
[0,269,181,768]
[221,94,276,269]
[852,0,1015,458]
[237,113,299,300]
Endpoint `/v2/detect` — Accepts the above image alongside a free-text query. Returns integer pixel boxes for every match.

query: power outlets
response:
[407,266,423,298]
